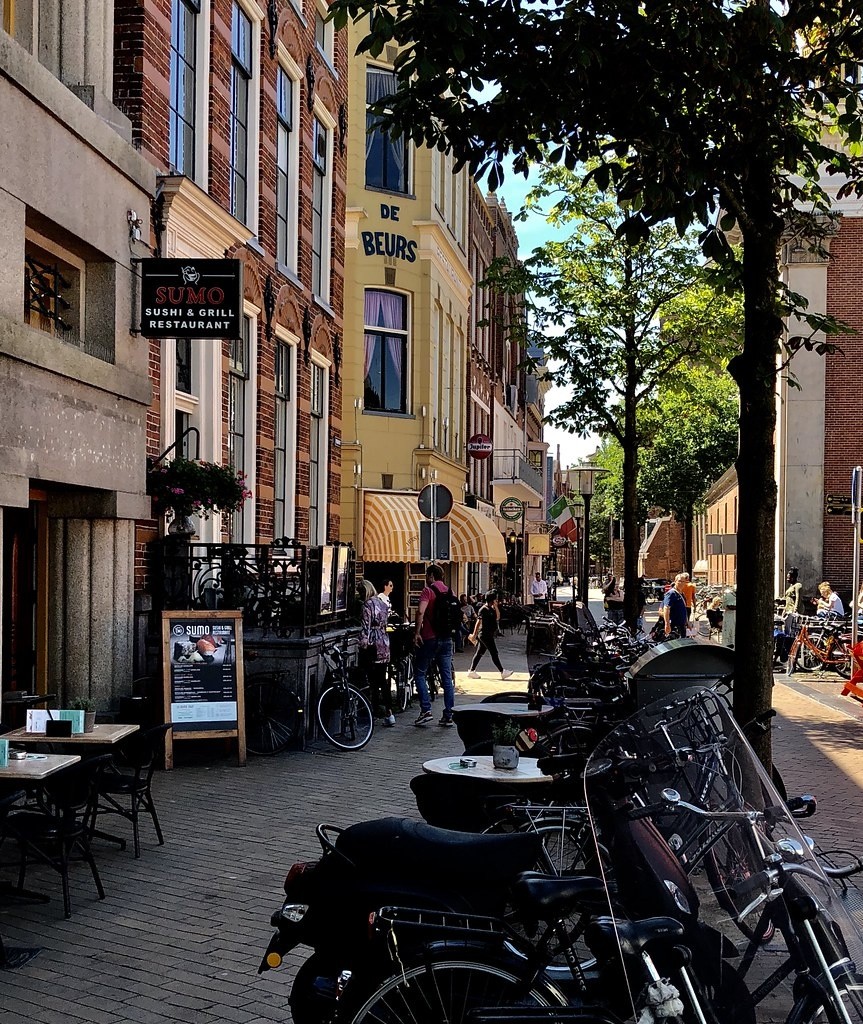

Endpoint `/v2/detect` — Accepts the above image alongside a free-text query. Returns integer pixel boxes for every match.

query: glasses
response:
[388,585,393,587]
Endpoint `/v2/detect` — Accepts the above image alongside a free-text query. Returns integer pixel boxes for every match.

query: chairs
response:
[409,772,527,835]
[460,740,493,755]
[706,609,723,641]
[451,709,524,749]
[0,722,175,918]
[480,692,547,705]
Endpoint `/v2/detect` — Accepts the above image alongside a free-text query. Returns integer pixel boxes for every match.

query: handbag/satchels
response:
[358,644,377,663]
[650,620,675,641]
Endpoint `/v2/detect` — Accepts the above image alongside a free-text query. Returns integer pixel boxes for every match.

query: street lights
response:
[569,503,585,601]
[570,460,610,609]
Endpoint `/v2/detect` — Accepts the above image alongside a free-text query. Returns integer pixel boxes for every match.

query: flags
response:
[548,495,579,542]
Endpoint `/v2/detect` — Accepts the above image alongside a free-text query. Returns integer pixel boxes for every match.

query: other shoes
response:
[456,648,464,653]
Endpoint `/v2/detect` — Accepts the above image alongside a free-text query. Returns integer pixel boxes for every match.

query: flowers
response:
[147,455,253,521]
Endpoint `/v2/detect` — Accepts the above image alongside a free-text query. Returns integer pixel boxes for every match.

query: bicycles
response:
[309,636,390,752]
[257,573,863,1024]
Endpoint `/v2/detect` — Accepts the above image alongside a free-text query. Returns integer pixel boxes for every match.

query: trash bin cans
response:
[624,637,735,841]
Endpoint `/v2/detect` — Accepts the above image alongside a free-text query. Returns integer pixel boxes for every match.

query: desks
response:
[450,703,554,718]
[422,755,554,783]
[0,753,82,959]
[0,723,141,851]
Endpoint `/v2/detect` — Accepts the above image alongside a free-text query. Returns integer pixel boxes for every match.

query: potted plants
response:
[68,696,98,733]
[490,718,521,768]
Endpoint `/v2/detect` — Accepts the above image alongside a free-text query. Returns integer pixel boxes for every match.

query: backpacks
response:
[425,585,463,638]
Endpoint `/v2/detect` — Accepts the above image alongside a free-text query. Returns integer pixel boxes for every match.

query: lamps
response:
[421,468,426,480]
[422,405,426,417]
[354,397,363,409]
[462,483,468,492]
[354,464,362,475]
[431,469,438,480]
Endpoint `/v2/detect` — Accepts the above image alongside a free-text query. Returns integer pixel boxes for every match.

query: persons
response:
[602,572,624,624]
[636,574,649,626]
[783,566,803,613]
[356,580,396,726]
[663,574,692,639]
[468,591,513,680]
[411,565,454,725]
[682,572,696,621]
[659,600,665,626]
[811,581,843,616]
[379,580,394,611]
[858,583,863,616]
[453,593,505,653]
[706,596,723,629]
[530,572,547,606]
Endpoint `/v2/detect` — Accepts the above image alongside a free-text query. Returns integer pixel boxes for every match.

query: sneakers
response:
[415,710,433,724]
[467,669,480,679]
[382,715,396,725]
[501,669,513,679]
[438,717,453,726]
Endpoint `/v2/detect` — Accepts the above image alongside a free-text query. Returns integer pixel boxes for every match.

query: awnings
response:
[363,492,507,564]
